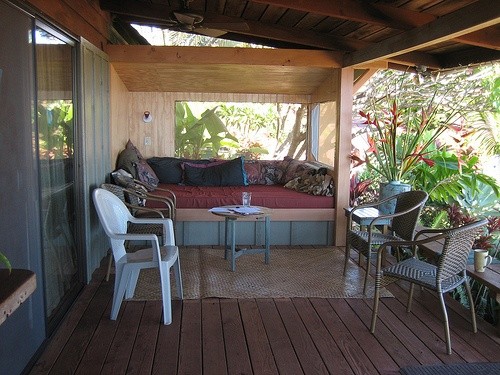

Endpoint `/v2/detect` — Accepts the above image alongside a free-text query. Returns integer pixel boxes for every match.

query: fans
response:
[102,0,249,38]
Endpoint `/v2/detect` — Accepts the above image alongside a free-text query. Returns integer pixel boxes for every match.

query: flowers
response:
[348,64,472,180]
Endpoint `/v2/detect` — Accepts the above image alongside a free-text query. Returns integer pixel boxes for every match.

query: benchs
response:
[413,226,500,291]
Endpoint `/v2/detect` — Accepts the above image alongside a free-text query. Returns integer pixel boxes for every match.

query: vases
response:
[379,181,411,215]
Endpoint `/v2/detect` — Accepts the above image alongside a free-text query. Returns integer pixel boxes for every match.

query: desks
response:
[344,207,390,268]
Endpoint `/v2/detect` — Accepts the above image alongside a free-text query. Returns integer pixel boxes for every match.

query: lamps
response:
[143,111,152,122]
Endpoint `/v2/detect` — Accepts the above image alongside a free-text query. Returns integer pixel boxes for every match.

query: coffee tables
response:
[211,205,273,271]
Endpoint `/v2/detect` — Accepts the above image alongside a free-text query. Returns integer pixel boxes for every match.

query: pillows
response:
[118,139,328,206]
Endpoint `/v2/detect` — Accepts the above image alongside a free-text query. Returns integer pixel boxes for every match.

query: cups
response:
[474,249,492,273]
[241,191,253,208]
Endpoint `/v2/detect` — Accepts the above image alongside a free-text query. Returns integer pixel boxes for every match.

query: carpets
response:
[131,247,395,300]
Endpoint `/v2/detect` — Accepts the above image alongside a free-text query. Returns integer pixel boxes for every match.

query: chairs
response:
[343,189,488,355]
[92,172,183,325]
[44,182,77,278]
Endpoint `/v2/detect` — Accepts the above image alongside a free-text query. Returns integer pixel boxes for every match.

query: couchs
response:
[115,156,335,246]
